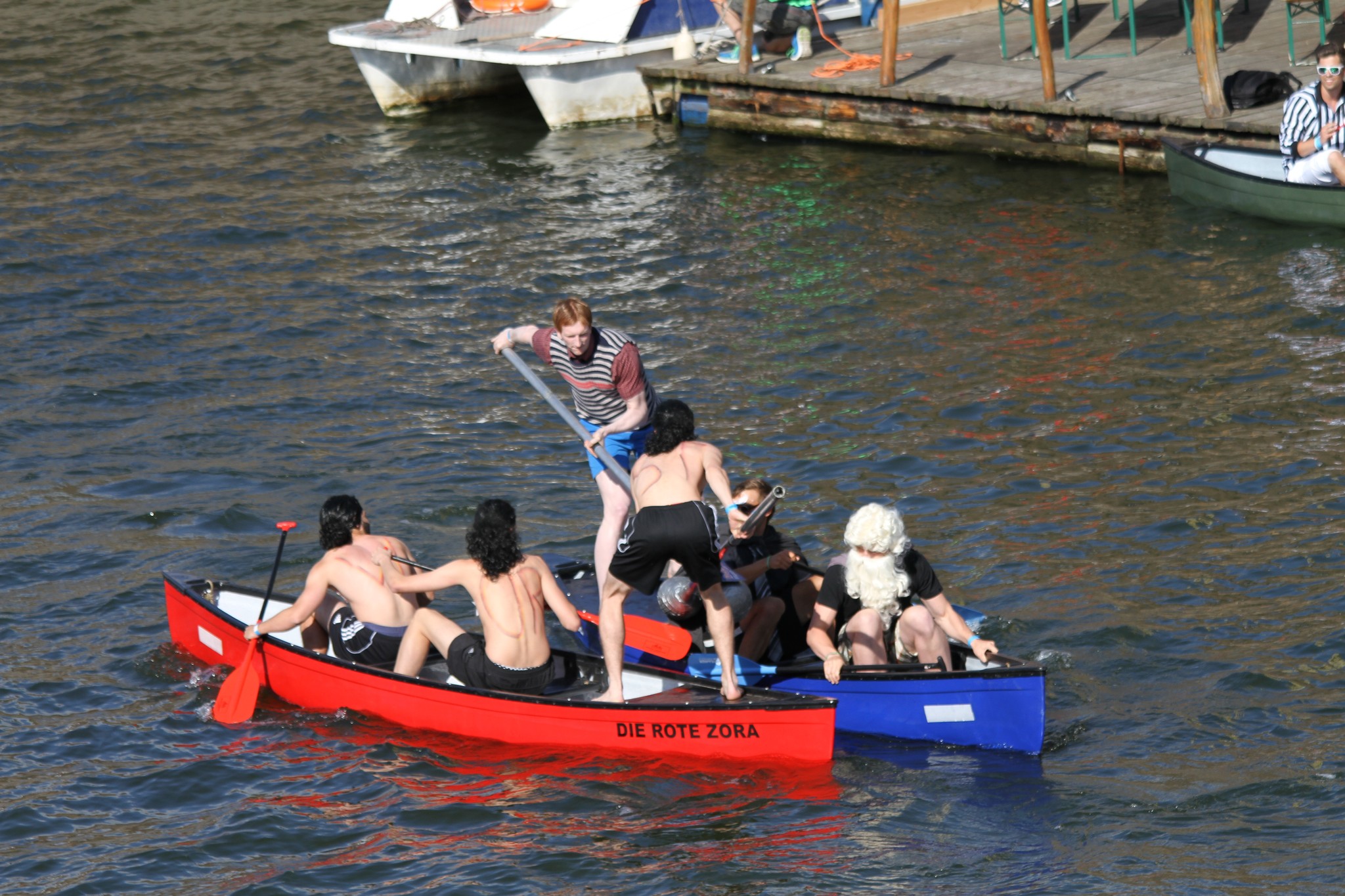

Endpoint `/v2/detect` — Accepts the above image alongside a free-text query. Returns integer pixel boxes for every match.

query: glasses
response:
[1317,65,1342,76]
[737,502,757,515]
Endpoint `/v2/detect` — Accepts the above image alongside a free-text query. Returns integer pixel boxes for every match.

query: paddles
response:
[389,550,693,664]
[211,522,297,726]
[787,557,987,638]
[684,656,946,689]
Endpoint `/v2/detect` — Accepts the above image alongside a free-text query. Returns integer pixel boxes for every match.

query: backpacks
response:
[1223,70,1301,111]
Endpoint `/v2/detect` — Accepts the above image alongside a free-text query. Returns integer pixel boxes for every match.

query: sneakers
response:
[787,25,813,60]
[716,44,761,64]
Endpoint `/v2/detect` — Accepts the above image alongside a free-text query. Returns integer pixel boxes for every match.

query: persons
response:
[590,398,744,703]
[705,0,818,64]
[1280,43,1345,187]
[805,502,998,684]
[705,479,837,666]
[244,495,435,665]
[490,298,684,613]
[373,500,582,697]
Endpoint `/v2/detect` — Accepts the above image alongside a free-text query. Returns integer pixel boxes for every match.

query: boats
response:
[327,0,1062,130]
[159,570,839,767]
[540,552,1047,758]
[1155,134,1345,226]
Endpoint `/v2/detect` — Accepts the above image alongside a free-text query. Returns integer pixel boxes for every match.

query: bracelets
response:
[507,329,516,345]
[823,652,840,658]
[254,624,265,637]
[1314,134,1323,152]
[766,555,772,569]
[725,505,739,514]
[968,635,980,649]
